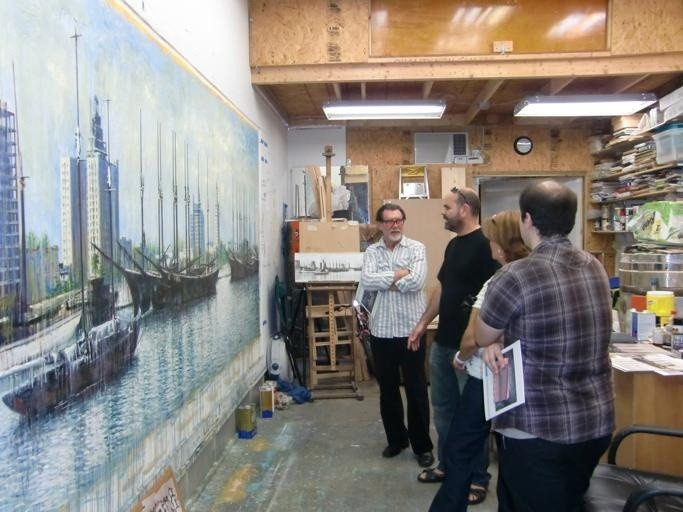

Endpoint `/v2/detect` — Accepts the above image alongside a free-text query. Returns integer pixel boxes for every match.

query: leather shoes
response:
[417,451,434,467]
[383,444,409,457]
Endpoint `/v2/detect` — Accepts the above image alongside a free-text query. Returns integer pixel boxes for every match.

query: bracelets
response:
[454,350,466,365]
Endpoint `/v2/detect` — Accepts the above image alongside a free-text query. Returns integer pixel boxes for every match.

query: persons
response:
[360,203,434,468]
[428,210,531,511]
[408,186,502,505]
[474,178,616,512]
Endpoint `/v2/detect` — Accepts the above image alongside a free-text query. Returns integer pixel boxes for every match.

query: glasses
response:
[450,186,468,205]
[382,218,404,229]
[491,214,497,225]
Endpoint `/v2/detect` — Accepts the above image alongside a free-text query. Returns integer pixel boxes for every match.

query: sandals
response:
[418,468,446,483]
[467,481,489,505]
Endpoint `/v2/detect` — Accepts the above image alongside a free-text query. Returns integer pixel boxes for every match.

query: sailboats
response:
[90,108,260,316]
[1,161,140,415]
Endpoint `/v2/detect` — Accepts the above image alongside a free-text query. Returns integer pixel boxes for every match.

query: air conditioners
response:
[414,132,469,165]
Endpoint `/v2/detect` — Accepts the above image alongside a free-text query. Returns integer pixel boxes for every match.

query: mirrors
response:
[290,165,370,224]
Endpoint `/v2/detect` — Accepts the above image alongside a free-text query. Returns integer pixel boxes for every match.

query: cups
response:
[653,328,665,344]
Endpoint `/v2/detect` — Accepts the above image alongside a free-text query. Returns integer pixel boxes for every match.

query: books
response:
[481,338,527,422]
[588,85,683,376]
[355,284,382,319]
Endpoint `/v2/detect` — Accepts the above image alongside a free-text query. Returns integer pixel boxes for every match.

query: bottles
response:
[671,325,683,350]
[669,310,679,345]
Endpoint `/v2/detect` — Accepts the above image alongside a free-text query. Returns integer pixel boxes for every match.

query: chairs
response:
[569,424,683,512]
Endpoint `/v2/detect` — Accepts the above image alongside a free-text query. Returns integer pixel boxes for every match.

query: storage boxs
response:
[619,245,683,296]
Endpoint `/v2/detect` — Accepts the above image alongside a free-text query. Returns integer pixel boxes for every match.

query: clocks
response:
[514,136,533,155]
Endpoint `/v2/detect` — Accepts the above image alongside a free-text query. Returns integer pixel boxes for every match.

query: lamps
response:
[322,98,446,121]
[514,93,657,117]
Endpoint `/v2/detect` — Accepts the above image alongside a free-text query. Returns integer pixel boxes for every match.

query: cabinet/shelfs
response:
[589,112,683,234]
[598,368,683,478]
